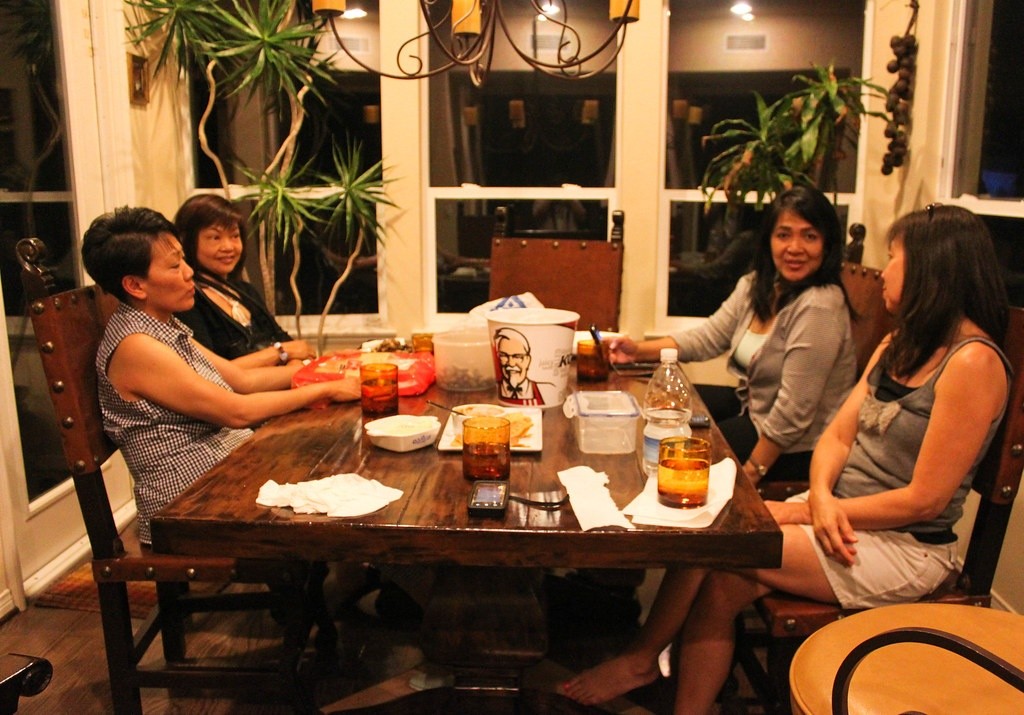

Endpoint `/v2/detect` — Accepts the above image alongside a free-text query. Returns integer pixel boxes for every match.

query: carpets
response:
[36,511,230,616]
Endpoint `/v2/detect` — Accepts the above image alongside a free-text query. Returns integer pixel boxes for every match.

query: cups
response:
[360,362,398,418]
[462,415,511,482]
[577,339,609,384]
[657,435,712,510]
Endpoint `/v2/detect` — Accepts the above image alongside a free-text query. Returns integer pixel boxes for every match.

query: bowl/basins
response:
[363,414,442,453]
[431,331,497,392]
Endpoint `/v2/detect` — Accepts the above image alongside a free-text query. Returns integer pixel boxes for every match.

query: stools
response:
[788,602,1024,714]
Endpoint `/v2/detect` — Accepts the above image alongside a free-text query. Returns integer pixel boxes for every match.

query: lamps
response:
[310,0,638,80]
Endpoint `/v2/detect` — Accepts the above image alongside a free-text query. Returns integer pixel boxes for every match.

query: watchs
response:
[747,456,767,476]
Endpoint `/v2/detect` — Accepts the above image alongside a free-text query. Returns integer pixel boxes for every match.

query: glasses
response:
[926,202,943,221]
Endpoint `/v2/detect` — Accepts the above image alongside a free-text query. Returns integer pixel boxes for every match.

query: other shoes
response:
[547,578,642,632]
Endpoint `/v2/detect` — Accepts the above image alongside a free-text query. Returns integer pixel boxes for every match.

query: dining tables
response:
[149,332,786,715]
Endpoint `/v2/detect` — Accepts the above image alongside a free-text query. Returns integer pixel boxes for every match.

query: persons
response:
[555,205,1014,715]
[78,207,395,667]
[172,194,319,366]
[565,187,859,587]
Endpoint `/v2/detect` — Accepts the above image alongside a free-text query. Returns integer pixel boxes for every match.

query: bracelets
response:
[271,341,288,363]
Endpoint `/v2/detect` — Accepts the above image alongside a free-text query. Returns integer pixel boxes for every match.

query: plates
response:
[437,404,543,451]
[573,330,623,355]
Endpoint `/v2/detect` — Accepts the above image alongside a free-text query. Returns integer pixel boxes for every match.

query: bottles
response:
[641,348,693,477]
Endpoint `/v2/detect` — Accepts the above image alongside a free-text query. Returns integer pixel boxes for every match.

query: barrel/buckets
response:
[483,309,580,409]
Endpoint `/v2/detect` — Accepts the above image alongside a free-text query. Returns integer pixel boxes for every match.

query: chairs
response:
[489,206,626,340]
[759,224,900,503]
[727,309,1024,715]
[17,237,340,715]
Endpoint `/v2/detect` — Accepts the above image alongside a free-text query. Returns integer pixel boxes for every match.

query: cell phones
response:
[466,480,510,518]
[689,415,710,427]
[590,323,602,344]
